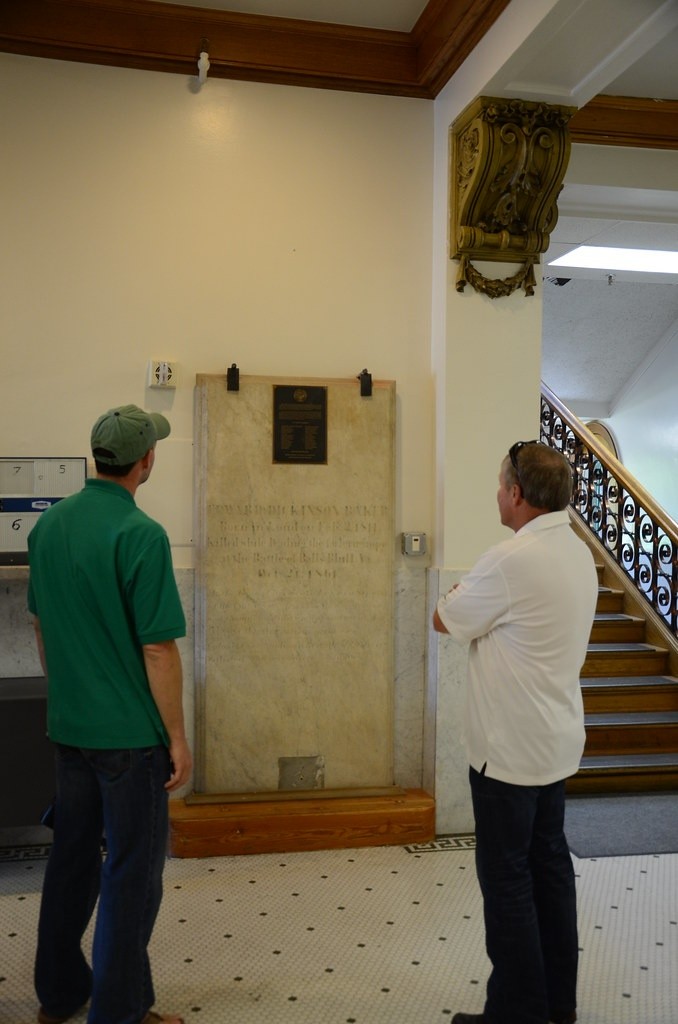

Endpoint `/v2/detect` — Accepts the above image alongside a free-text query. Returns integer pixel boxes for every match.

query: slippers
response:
[142,1009,185,1024]
[36,1014,66,1024]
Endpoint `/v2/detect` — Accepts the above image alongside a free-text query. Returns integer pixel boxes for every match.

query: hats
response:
[91,403,170,466]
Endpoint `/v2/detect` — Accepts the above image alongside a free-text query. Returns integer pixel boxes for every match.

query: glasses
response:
[508,439,548,473]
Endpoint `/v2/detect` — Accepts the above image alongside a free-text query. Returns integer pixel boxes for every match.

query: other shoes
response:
[449,1011,486,1024]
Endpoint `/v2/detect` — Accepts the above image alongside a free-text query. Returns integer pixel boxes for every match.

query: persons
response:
[431,437,602,1023]
[26,405,196,1023]
